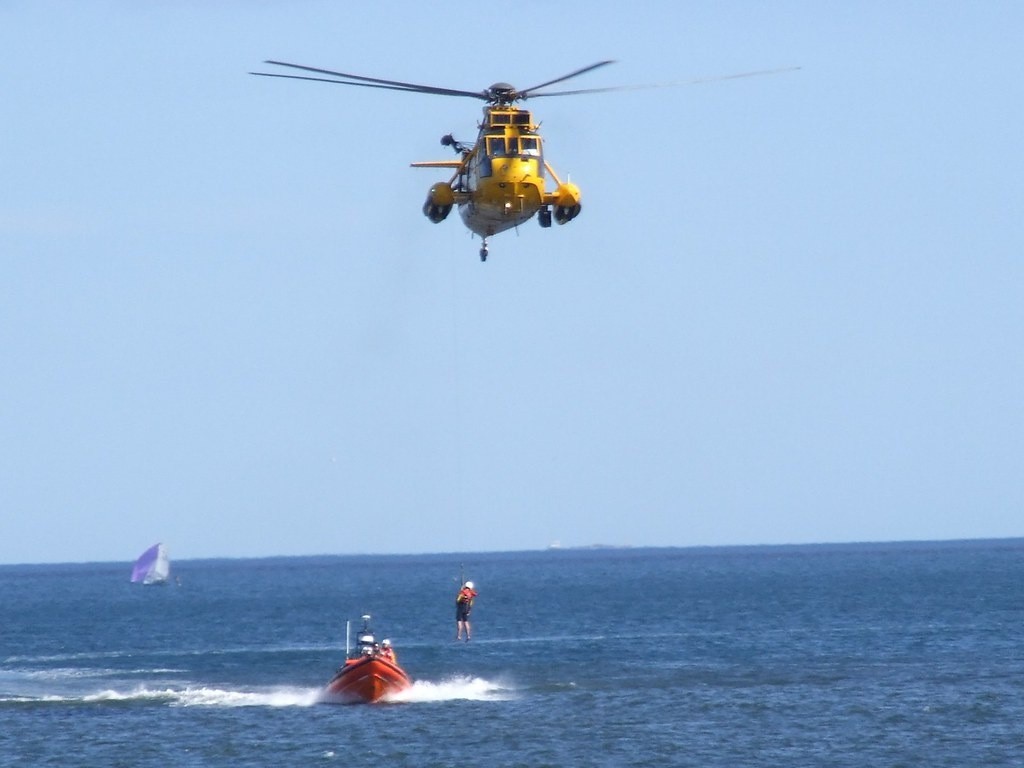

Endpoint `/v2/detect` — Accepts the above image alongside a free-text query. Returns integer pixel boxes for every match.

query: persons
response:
[371,638,396,664]
[455,581,477,641]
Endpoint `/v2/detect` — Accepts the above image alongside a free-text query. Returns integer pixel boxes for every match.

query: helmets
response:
[382,639,391,645]
[465,581,473,589]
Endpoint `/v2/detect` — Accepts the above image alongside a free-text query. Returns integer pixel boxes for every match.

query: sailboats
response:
[126,541,170,587]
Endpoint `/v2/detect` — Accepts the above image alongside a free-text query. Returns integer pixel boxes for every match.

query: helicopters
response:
[250,54,809,263]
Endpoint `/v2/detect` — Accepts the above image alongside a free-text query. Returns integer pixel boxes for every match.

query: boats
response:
[322,615,415,703]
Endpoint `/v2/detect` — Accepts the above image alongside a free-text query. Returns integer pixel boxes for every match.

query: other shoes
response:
[465,635,471,643]
[457,636,462,640]
